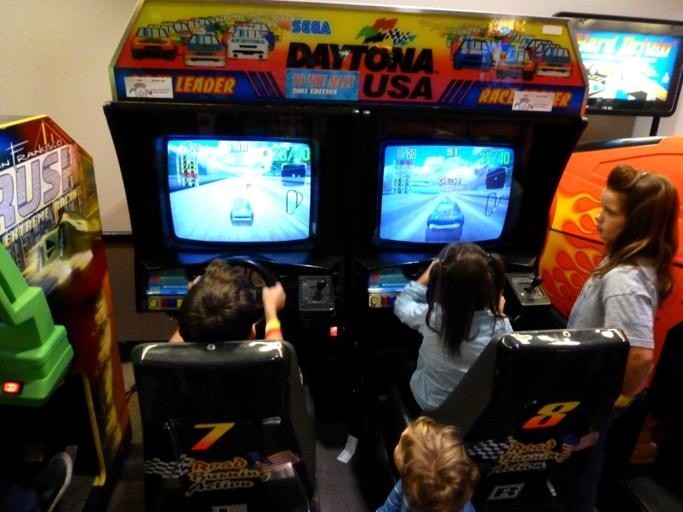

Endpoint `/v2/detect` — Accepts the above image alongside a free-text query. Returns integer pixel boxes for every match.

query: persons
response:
[376,417,482,511]
[394,243,516,411]
[167,257,292,342]
[566,164,681,511]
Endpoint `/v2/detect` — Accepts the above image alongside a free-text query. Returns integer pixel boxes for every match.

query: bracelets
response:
[263,319,283,331]
[264,328,282,338]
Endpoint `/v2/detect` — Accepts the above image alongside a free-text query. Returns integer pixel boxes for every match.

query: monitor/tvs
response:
[375,139,519,254]
[154,133,321,253]
[553,12,683,116]
